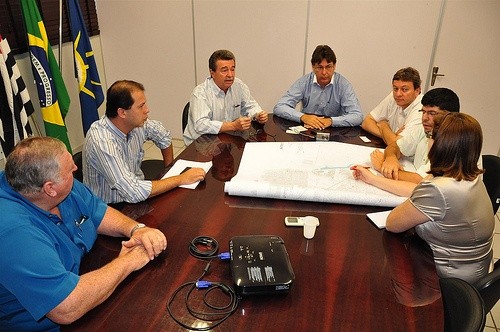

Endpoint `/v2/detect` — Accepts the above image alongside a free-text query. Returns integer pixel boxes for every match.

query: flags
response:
[0,28,35,159]
[64,0,105,135]
[21,0,73,157]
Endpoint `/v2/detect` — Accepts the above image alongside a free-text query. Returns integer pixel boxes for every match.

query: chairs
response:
[141,159,164,179]
[482,155,500,215]
[72,152,83,182]
[182,102,189,134]
[440,262,500,332]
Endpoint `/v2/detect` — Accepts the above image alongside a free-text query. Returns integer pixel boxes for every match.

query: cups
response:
[303,216,319,239]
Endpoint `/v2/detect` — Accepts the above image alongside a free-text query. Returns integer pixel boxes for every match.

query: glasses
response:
[314,65,334,72]
[426,132,432,138]
[418,110,439,118]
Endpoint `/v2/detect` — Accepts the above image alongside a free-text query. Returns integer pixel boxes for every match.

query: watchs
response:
[130,223,146,236]
[330,118,332,122]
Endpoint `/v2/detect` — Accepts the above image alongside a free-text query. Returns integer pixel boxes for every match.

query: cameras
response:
[316,133,330,141]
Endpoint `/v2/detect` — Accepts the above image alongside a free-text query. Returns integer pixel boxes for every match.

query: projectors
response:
[229,235,295,298]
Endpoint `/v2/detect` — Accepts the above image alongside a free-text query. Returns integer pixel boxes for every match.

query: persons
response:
[183,50,268,147]
[273,45,363,130]
[362,67,424,161]
[350,112,495,284]
[0,137,167,332]
[370,87,484,184]
[82,80,206,204]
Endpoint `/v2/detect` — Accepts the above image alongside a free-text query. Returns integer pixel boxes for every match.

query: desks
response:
[61,112,444,332]
